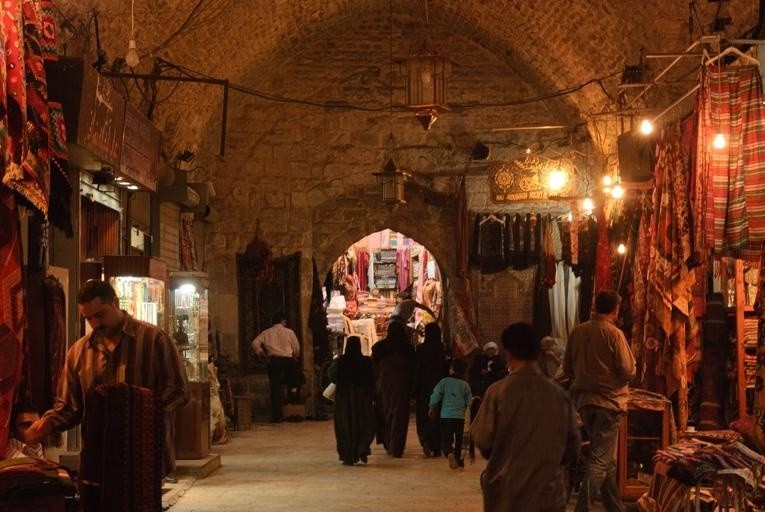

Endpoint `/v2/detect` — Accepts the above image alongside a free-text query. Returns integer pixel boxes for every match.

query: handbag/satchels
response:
[322,383,336,403]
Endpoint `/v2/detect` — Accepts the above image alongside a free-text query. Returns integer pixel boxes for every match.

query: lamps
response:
[178,150,195,169]
[125,0,141,66]
[711,17,731,32]
[619,45,700,88]
[369,1,454,216]
[473,141,490,159]
[95,161,115,185]
[712,33,729,150]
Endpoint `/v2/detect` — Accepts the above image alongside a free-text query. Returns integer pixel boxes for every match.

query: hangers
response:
[478,211,505,227]
[704,36,760,69]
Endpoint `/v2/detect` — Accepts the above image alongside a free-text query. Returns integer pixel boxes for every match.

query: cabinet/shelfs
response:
[615,396,672,502]
[723,256,765,427]
[370,247,399,290]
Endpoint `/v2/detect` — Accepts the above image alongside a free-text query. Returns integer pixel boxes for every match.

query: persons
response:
[5,402,70,480]
[250,313,301,422]
[326,289,509,469]
[471,322,582,512]
[563,290,637,511]
[24,279,192,512]
[541,333,566,385]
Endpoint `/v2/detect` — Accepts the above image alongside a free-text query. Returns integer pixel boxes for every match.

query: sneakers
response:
[448,453,458,468]
[424,440,441,457]
